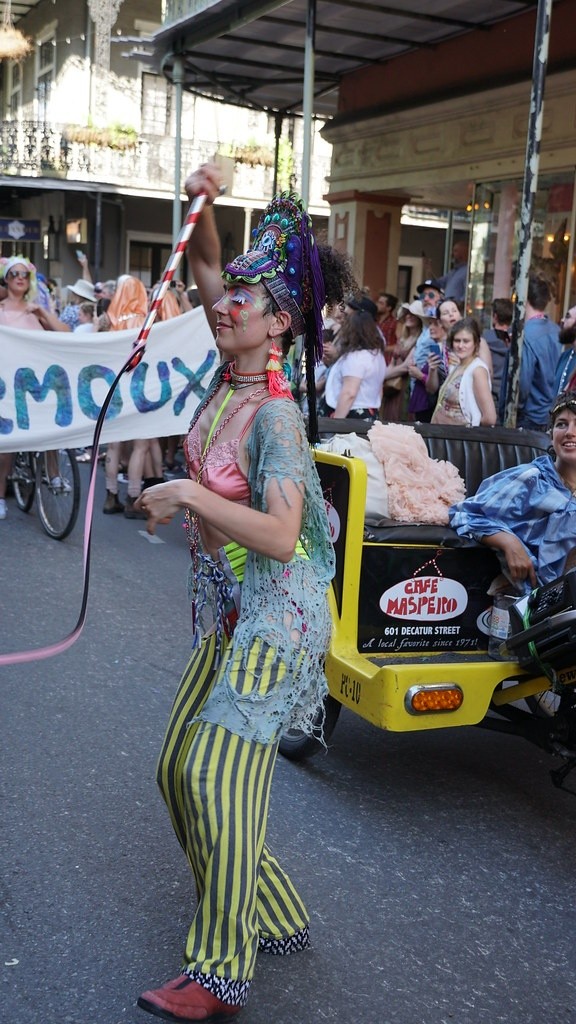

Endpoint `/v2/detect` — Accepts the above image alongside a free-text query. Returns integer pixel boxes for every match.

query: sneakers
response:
[51,476,72,492]
[0,499,8,520]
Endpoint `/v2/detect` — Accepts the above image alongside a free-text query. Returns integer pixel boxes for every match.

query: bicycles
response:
[6,448,81,541]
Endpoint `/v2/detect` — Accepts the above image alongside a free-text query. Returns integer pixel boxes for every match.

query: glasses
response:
[9,270,31,279]
[420,292,440,299]
[551,397,576,416]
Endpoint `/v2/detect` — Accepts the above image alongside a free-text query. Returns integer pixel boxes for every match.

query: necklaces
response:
[228,378,255,390]
[229,360,268,384]
[183,367,269,637]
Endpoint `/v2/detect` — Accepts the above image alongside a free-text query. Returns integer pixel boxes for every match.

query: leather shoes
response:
[137,975,243,1024]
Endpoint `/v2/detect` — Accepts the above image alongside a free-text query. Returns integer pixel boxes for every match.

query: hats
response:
[67,279,97,303]
[421,306,438,318]
[338,295,379,322]
[417,279,444,296]
[402,301,424,321]
[0,254,37,303]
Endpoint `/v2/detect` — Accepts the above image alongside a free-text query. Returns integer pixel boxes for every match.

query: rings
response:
[218,185,226,195]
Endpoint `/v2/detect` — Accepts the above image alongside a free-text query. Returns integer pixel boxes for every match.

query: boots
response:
[124,494,148,520]
[102,489,125,513]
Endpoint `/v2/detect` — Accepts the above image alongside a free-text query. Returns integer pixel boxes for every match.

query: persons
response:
[449,389,576,719]
[131,163,360,1024]
[0,241,576,525]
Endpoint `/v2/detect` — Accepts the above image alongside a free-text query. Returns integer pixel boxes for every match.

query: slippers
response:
[75,453,105,463]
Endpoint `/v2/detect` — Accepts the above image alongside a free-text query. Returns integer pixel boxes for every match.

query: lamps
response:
[466,201,490,211]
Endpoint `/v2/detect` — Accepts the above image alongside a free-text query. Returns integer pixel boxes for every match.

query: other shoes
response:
[117,472,145,485]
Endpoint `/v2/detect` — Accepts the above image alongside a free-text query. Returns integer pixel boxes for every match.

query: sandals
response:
[161,460,182,471]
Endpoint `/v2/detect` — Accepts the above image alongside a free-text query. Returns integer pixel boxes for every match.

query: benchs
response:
[307,420,556,654]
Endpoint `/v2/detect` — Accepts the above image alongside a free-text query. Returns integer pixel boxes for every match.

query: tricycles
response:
[277,418,576,798]
